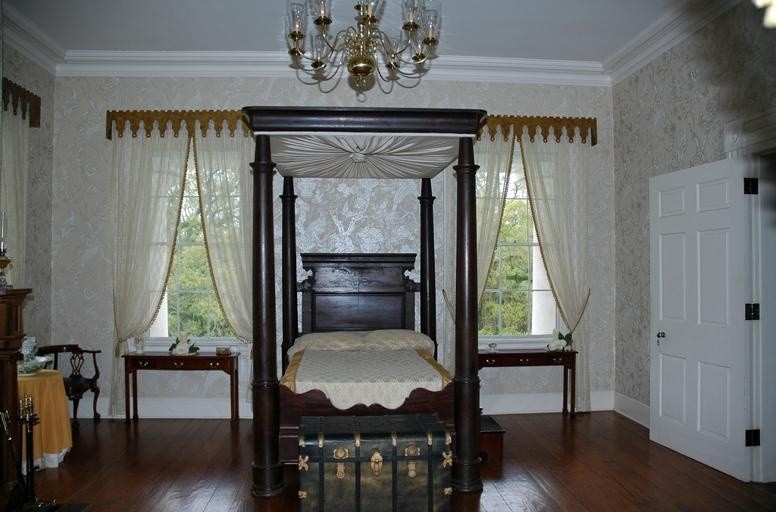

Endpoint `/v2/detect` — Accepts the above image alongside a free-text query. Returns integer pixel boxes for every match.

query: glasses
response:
[284,0,441,92]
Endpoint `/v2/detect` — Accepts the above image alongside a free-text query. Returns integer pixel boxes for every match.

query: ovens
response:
[242,104,486,499]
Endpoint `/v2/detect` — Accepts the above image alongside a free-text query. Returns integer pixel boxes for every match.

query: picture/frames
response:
[17,370,73,476]
[121,350,240,421]
[479,343,577,418]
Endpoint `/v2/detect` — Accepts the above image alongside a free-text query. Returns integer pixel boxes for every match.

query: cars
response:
[216,341,230,356]
[136,341,144,353]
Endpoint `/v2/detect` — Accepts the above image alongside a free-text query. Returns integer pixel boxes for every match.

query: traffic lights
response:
[286,328,435,354]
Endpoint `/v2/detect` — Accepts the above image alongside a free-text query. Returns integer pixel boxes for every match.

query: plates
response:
[0,286,32,511]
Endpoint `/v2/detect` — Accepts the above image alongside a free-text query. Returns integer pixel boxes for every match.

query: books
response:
[296,413,454,512]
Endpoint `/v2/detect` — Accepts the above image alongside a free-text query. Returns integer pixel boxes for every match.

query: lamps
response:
[35,345,102,426]
